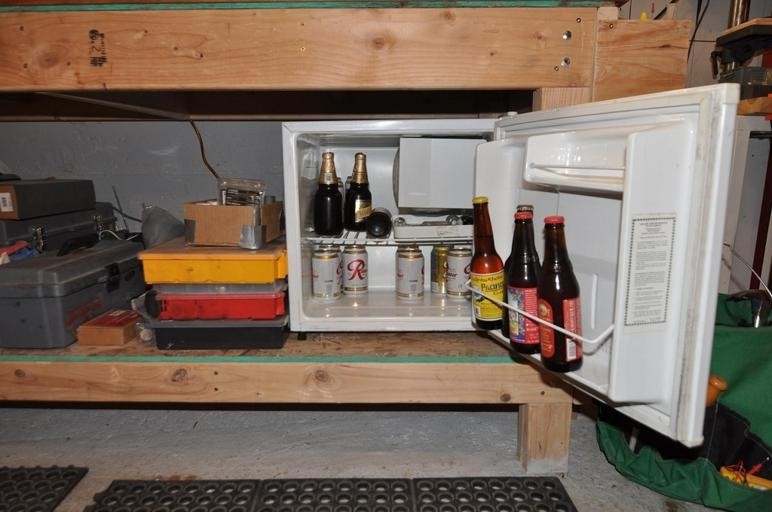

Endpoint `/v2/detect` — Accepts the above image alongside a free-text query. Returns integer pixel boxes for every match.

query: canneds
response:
[311,244,368,301]
[394,244,473,301]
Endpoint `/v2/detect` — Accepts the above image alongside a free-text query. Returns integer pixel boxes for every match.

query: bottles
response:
[469,195,505,331]
[535,215,585,373]
[313,151,343,235]
[343,152,373,232]
[506,203,544,355]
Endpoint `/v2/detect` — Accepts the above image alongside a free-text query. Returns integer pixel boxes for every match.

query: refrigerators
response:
[280,81,742,451]
